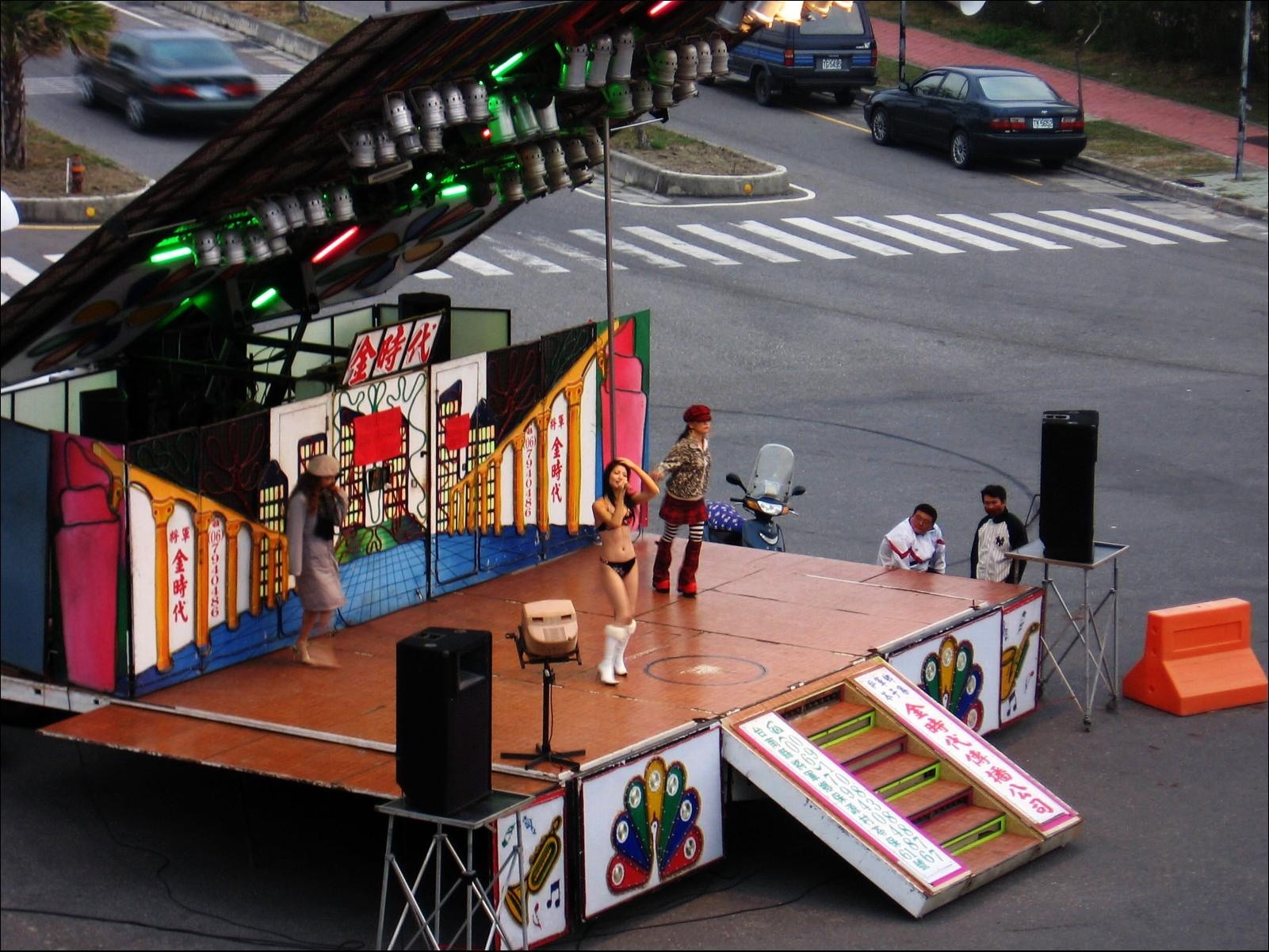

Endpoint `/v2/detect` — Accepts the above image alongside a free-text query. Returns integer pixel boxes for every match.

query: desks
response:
[1004,538,1129,733]
[375,785,536,952]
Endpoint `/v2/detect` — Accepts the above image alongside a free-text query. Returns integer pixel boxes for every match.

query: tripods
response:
[504,622,586,775]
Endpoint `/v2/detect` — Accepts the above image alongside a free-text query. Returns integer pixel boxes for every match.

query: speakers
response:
[396,625,493,817]
[1040,407,1099,562]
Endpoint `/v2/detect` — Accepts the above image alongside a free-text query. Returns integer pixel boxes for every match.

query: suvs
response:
[717,0,879,110]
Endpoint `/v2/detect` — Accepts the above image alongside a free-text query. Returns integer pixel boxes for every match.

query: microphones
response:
[624,480,632,488]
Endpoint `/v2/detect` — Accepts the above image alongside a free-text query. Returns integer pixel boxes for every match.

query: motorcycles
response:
[703,443,807,553]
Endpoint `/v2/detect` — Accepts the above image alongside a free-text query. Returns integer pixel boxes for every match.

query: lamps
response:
[190,0,853,268]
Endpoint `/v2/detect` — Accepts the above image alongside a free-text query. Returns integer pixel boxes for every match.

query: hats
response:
[683,405,712,421]
[309,455,339,478]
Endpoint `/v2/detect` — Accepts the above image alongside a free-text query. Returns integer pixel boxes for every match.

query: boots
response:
[614,619,636,675]
[596,624,626,684]
[653,538,673,593]
[678,541,702,595]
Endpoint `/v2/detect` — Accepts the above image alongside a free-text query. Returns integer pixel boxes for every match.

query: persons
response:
[876,503,947,575]
[592,457,660,684]
[285,454,347,669]
[650,405,713,596]
[970,485,1029,584]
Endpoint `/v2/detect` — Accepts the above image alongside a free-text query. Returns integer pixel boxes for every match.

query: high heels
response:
[312,642,340,667]
[292,641,315,665]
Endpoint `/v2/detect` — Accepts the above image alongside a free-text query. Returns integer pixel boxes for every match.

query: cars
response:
[72,29,262,138]
[862,66,1088,170]
